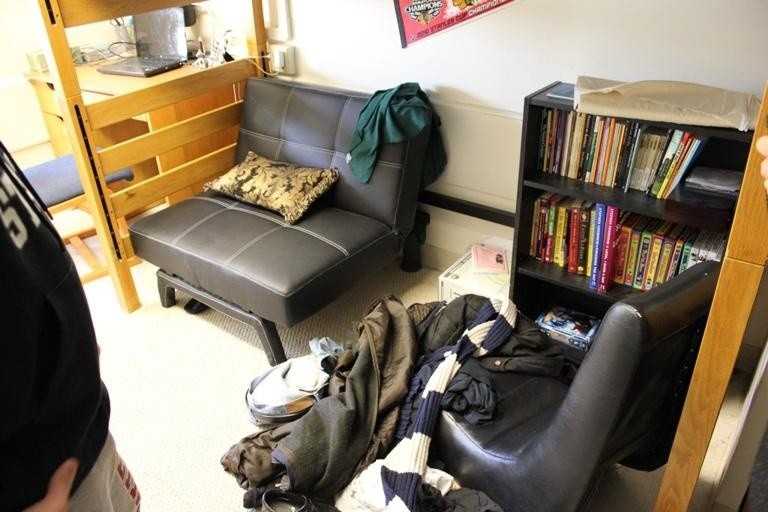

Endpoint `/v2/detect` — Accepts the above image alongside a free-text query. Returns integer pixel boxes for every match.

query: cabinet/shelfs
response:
[508,80,755,436]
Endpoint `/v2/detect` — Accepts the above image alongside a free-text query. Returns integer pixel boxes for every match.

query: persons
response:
[0,138,141,512]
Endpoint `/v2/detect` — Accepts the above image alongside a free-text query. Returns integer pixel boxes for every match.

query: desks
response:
[22,43,249,208]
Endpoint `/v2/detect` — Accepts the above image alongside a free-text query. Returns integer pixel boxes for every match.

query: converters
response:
[273,51,284,68]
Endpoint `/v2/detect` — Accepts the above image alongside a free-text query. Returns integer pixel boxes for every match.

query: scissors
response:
[109,15,125,27]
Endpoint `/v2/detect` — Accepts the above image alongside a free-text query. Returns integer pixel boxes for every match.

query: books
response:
[471,242,508,275]
[530,191,726,294]
[528,105,707,200]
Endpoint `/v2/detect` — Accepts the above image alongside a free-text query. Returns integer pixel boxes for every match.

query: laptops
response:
[96,8,188,78]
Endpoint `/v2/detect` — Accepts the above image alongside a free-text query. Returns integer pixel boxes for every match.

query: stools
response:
[17,145,134,283]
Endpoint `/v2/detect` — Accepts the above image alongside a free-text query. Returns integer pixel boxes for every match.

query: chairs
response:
[433,261,722,511]
[127,76,431,371]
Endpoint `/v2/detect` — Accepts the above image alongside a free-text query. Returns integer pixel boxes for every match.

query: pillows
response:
[202,150,340,225]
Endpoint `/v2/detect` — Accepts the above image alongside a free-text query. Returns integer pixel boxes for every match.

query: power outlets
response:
[270,43,297,75]
